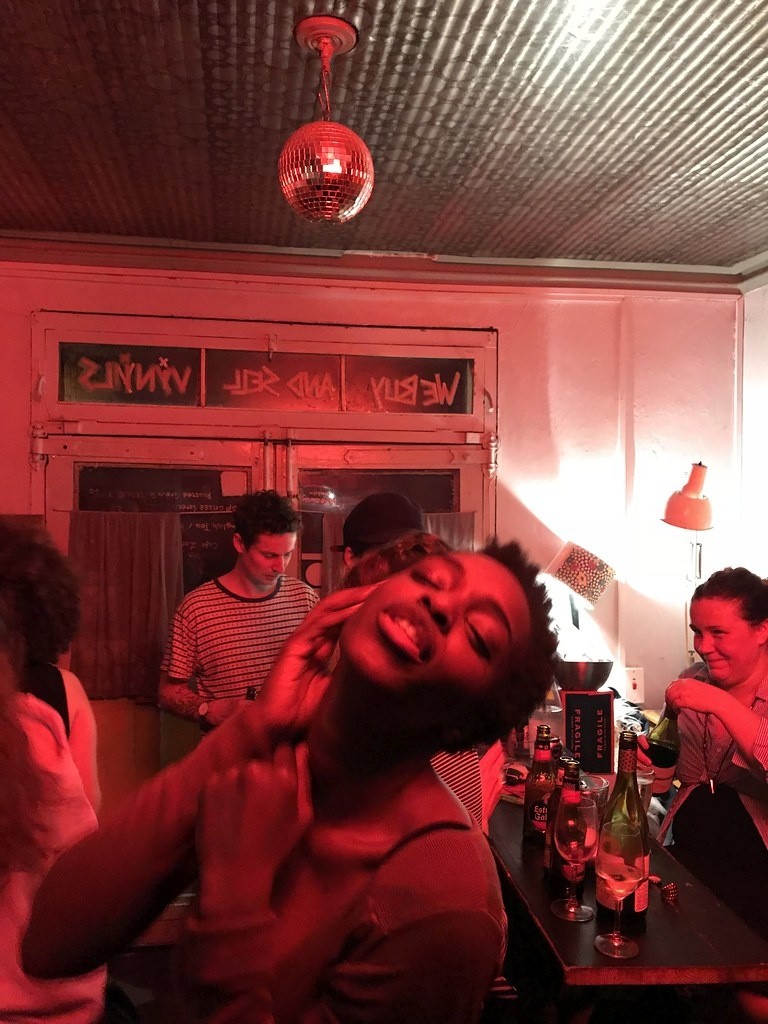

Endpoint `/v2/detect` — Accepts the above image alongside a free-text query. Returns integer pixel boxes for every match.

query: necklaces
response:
[695,679,758,797]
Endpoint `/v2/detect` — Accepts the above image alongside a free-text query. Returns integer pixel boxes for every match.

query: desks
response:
[491,799,768,984]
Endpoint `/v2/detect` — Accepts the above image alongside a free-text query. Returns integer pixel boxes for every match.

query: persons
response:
[0,511,108,1023]
[342,492,424,587]
[157,487,320,743]
[20,535,560,1024]
[636,567,768,949]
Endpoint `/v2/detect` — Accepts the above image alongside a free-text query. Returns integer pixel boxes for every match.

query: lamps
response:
[277,15,379,227]
[662,463,714,595]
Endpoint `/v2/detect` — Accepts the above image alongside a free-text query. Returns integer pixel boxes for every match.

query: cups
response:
[580,775,610,824]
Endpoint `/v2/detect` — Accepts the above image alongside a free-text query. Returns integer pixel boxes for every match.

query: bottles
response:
[594,730,649,938]
[504,716,580,889]
[648,704,682,804]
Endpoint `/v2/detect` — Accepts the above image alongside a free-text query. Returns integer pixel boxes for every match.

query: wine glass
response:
[594,821,640,961]
[550,796,599,921]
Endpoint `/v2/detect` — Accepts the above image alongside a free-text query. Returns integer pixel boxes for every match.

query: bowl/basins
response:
[552,661,613,691]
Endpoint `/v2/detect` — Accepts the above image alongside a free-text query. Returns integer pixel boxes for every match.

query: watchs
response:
[199,697,218,728]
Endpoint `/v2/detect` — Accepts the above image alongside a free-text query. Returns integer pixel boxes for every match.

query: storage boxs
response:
[556,690,614,776]
